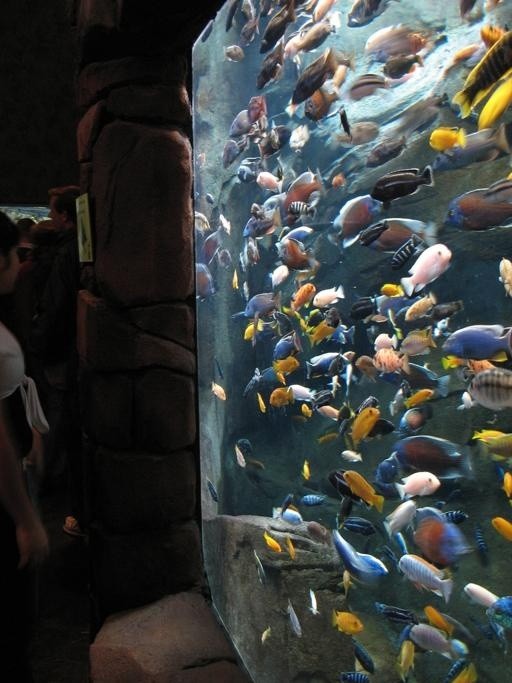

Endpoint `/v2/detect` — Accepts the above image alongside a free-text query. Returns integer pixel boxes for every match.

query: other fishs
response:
[191,1,511,681]
